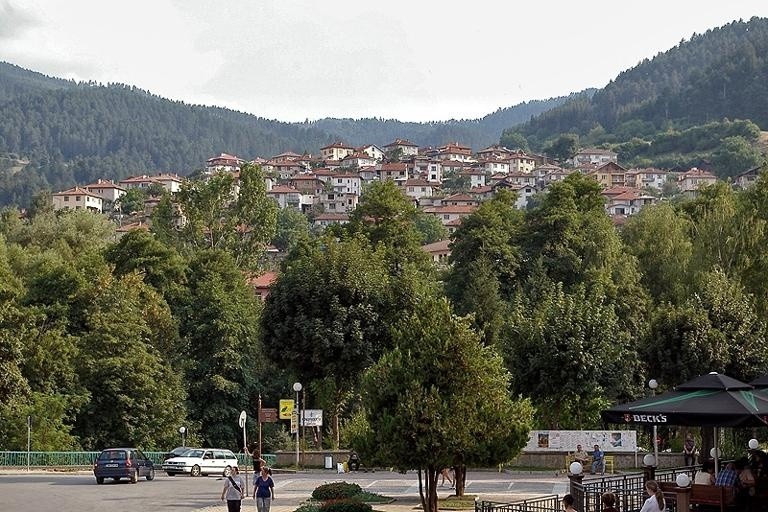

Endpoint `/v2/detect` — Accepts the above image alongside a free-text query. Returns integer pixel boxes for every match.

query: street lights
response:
[292,382,303,466]
[648,379,658,468]
[179,426,188,446]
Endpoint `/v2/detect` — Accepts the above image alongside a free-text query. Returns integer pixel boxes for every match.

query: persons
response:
[252,467,275,512]
[601,491,619,512]
[438,468,452,487]
[449,467,457,489]
[220,465,245,512]
[560,494,578,512]
[639,480,666,512]
[574,444,588,466]
[243,446,267,487]
[693,448,768,506]
[683,434,695,469]
[589,444,606,476]
[347,448,361,474]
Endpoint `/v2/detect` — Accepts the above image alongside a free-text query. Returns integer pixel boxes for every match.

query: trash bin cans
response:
[323,454,334,469]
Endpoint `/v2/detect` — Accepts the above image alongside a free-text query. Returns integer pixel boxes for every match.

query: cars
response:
[161,447,239,476]
[92,447,154,482]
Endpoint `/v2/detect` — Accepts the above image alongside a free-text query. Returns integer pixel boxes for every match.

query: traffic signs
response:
[259,408,278,423]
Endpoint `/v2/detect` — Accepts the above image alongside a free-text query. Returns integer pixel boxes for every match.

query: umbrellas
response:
[599,371,768,479]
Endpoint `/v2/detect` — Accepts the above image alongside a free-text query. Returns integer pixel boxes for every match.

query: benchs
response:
[658,481,737,512]
[565,453,615,477]
